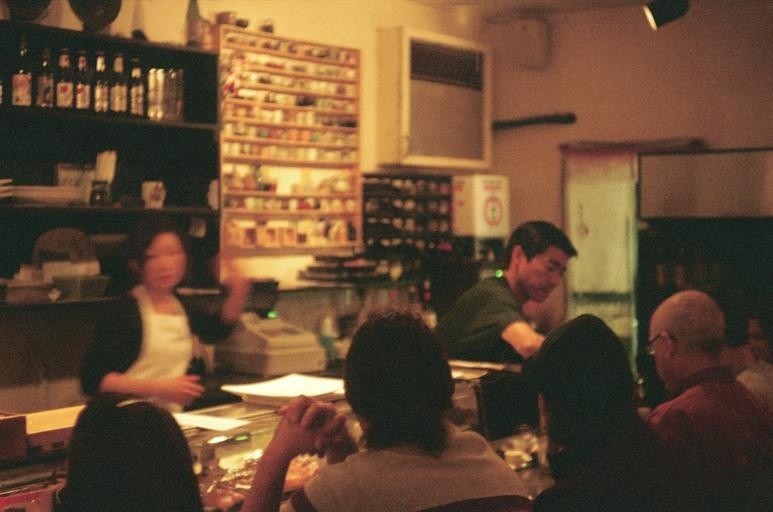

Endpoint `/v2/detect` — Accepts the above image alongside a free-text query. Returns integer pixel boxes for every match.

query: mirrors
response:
[399,27,494,171]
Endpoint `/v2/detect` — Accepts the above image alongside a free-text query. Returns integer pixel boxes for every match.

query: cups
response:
[141,180,167,209]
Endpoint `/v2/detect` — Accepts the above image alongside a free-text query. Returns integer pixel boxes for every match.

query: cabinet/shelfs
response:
[0,17,224,310]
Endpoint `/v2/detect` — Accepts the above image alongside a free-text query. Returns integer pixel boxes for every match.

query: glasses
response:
[644,332,677,357]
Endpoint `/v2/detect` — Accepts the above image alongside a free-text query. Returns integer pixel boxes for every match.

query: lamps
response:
[642,0,690,32]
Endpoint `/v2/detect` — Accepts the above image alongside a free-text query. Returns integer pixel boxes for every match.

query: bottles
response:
[86,178,111,206]
[10,33,146,119]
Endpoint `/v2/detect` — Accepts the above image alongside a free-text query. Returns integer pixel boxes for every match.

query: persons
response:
[747,298,773,364]
[36,390,208,512]
[237,310,535,512]
[76,222,256,406]
[645,288,773,512]
[433,219,578,363]
[717,309,773,416]
[517,315,694,512]
